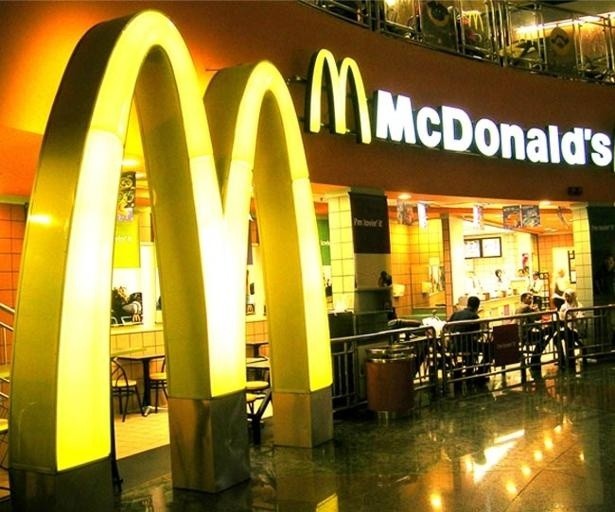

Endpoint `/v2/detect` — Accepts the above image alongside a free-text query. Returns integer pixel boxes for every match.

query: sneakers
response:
[480,377,490,381]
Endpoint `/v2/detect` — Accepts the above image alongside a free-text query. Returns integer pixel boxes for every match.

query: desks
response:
[119,353,166,416]
[246,339,270,421]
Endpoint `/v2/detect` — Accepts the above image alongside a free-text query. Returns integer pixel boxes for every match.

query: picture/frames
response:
[463,237,502,259]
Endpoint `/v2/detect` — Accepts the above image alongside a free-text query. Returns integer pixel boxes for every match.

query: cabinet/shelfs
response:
[354,285,395,401]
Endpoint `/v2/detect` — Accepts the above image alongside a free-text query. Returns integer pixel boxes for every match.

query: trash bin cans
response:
[364,343,418,424]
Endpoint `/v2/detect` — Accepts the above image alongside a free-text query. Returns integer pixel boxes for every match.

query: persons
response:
[557,290,588,364]
[112,288,141,322]
[493,269,506,290]
[517,293,547,364]
[446,296,494,382]
[530,272,544,312]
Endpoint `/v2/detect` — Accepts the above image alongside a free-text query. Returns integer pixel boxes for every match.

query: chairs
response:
[110,359,144,422]
[150,353,169,413]
[492,324,527,382]
